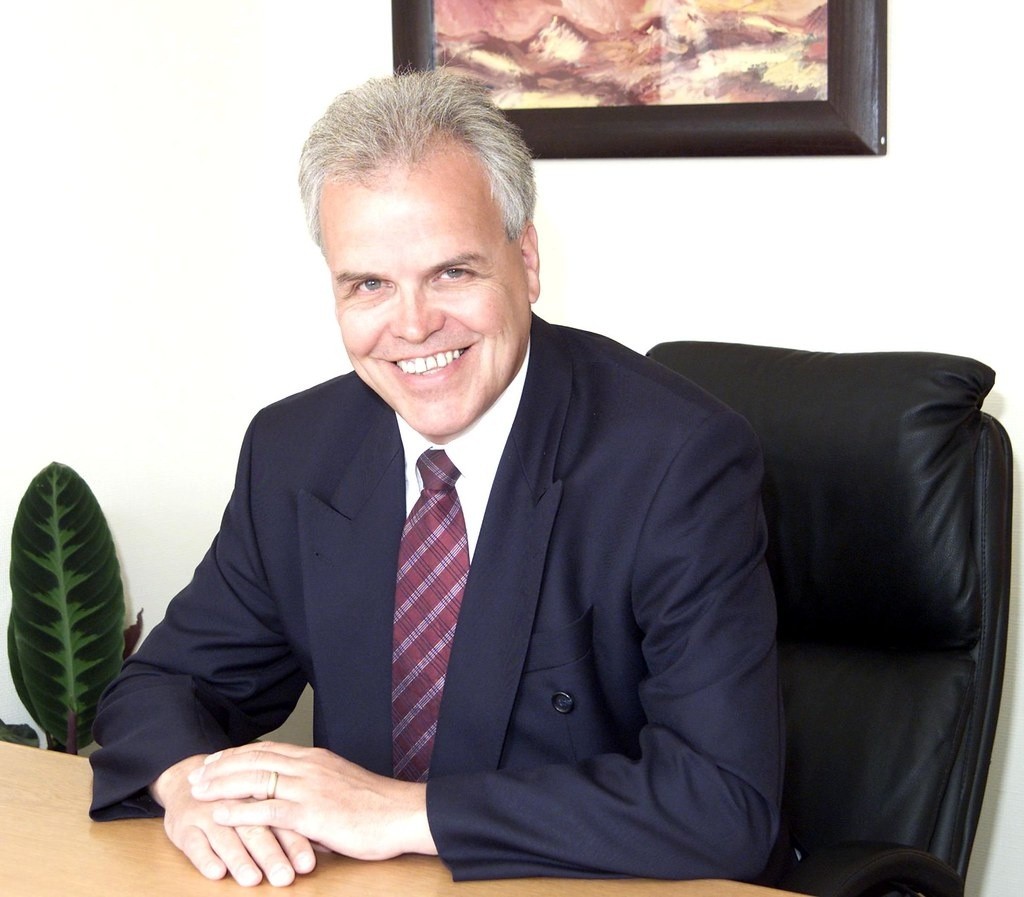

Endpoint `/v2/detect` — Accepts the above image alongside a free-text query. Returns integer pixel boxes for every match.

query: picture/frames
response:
[390,1,887,157]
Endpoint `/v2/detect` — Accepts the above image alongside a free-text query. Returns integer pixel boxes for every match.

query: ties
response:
[391,450,470,782]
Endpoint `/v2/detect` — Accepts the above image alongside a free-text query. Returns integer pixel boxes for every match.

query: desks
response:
[0,735,829,897]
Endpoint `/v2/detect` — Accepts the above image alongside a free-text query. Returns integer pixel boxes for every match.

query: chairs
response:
[624,341,1024,897]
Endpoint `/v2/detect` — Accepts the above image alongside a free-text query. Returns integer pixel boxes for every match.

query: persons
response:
[88,70,785,888]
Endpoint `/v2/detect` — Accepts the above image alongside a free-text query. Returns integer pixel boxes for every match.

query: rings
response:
[268,772,277,800]
